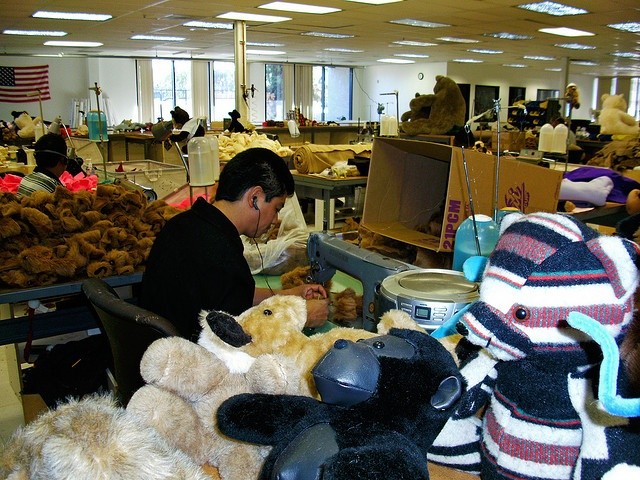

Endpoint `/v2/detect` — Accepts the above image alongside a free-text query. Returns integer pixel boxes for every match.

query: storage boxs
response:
[492,131,525,152]
[84,159,188,200]
[64,136,103,163]
[358,136,564,253]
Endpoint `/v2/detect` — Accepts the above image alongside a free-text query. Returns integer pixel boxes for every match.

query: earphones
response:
[252,196,276,296]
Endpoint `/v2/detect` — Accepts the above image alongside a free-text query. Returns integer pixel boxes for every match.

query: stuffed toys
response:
[401,75,466,135]
[427,212,640,480]
[0,390,214,480]
[198,295,429,401]
[228,110,244,133]
[126,337,300,480]
[598,94,640,135]
[169,106,205,155]
[217,329,467,480]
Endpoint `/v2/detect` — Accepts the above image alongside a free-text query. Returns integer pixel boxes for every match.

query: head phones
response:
[32,149,70,166]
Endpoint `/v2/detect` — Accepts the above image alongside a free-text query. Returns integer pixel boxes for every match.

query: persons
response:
[137,148,331,343]
[17,133,69,199]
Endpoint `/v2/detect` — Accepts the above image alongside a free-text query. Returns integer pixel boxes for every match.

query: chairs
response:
[81,277,179,411]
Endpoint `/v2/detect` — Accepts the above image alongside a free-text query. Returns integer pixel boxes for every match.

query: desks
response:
[1,272,150,305]
[250,270,363,336]
[124,123,378,161]
[288,168,367,231]
[107,130,125,160]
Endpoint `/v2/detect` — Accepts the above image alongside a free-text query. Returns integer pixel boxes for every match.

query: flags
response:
[0,64,51,103]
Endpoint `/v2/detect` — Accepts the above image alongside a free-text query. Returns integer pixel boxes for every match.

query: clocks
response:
[418,73,423,80]
